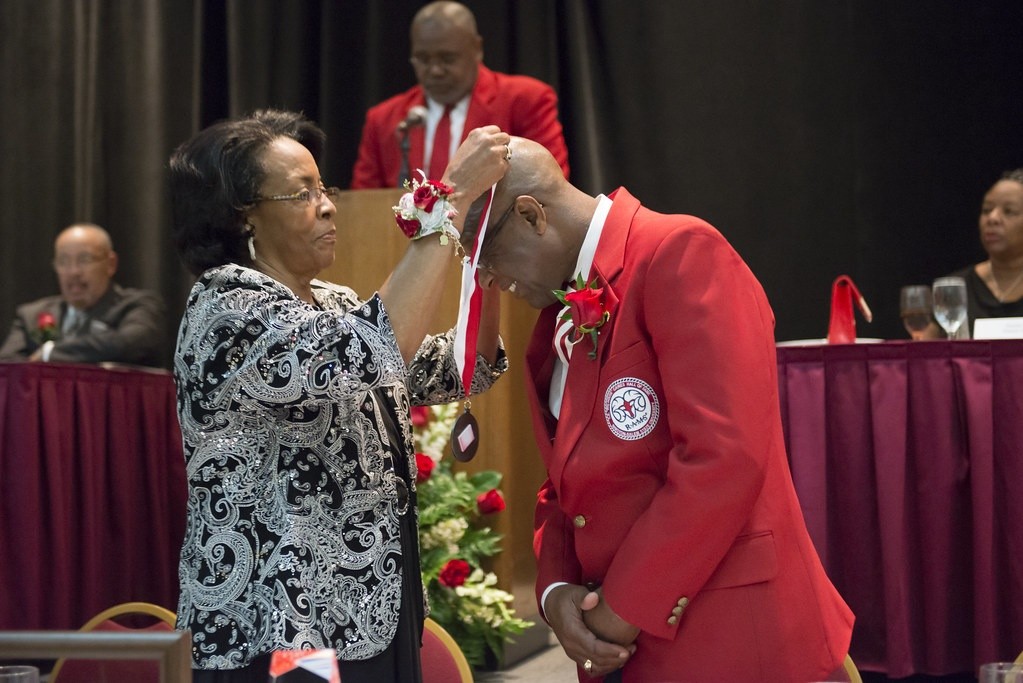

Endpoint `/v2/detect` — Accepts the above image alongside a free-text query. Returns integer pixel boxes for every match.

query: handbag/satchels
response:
[827,275,872,344]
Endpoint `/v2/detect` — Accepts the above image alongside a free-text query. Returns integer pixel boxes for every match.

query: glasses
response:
[473,198,545,269]
[255,187,340,206]
[53,253,110,269]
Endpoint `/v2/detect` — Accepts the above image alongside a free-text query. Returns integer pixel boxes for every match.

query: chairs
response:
[413,615,473,683]
[47,601,194,683]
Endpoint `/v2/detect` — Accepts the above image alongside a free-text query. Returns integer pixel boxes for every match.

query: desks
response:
[0,359,192,677]
[0,631,192,683]
[771,337,1022,680]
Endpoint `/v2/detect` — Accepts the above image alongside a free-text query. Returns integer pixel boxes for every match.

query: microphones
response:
[398,105,429,131]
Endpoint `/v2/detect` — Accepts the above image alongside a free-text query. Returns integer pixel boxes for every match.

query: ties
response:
[552,280,574,367]
[428,105,455,181]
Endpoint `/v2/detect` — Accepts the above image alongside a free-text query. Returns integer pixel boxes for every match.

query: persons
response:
[921,169,1023,339]
[352,2,569,191]
[0,223,168,363]
[458,135,863,683]
[161,108,514,683]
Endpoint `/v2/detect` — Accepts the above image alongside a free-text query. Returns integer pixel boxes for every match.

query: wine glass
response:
[901,276,969,341]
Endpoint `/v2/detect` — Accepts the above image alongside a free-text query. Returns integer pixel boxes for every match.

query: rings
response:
[583,659,592,673]
[504,145,512,161]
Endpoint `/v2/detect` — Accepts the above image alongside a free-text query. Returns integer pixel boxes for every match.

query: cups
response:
[979,662,1023,683]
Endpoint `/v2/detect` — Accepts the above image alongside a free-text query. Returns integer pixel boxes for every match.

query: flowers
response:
[33,310,63,340]
[550,270,613,361]
[408,403,536,669]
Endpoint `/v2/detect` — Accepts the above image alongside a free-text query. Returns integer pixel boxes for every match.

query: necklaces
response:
[988,260,1023,303]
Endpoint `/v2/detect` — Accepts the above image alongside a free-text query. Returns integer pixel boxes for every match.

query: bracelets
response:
[392,167,466,259]
